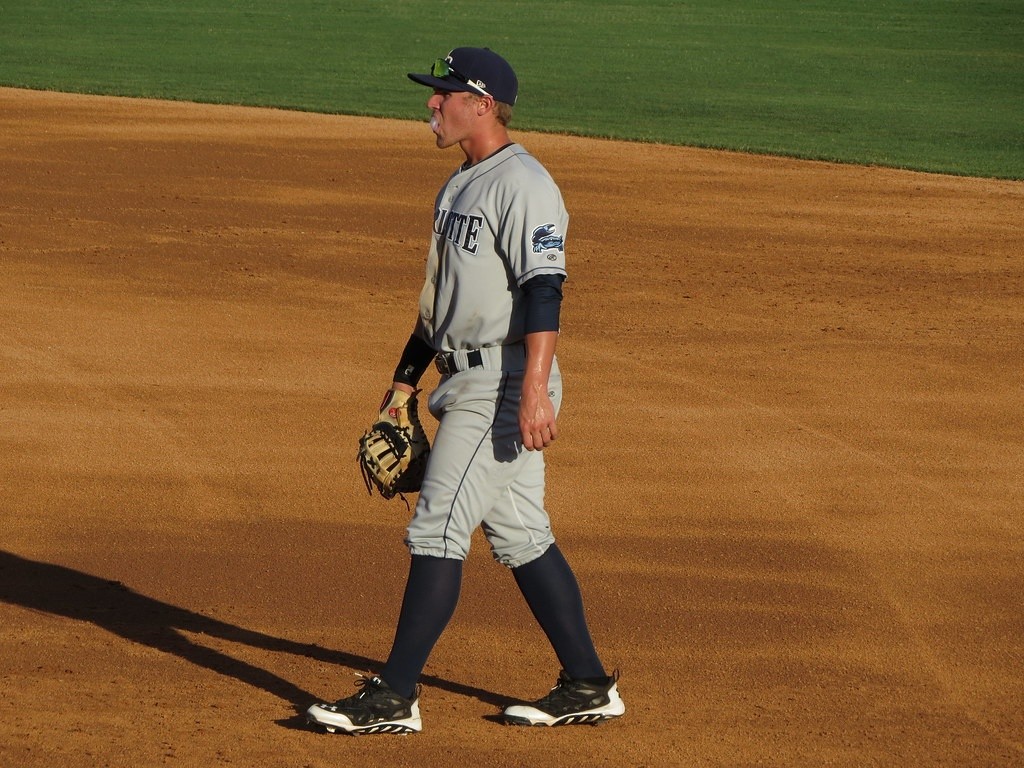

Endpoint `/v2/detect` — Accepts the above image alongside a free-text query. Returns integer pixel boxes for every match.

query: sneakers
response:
[306,675,422,736]
[502,668,626,726]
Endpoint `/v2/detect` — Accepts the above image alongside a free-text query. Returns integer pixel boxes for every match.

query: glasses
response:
[431,58,494,100]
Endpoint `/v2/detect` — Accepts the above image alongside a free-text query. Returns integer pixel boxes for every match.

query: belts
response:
[435,343,526,375]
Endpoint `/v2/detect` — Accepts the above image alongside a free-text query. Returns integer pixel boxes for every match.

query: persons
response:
[305,48,625,737]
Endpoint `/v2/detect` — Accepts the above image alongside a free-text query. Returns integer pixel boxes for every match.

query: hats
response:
[407,47,518,106]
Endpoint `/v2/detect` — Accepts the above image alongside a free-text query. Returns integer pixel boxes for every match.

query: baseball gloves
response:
[350,389,433,508]
